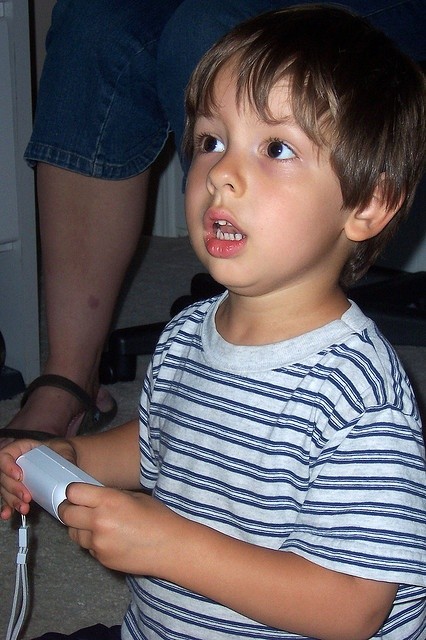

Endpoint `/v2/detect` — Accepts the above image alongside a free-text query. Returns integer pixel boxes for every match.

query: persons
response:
[2,1,276,444]
[1,7,425,639]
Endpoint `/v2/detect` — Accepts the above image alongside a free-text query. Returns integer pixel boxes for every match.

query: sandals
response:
[1,374,117,466]
